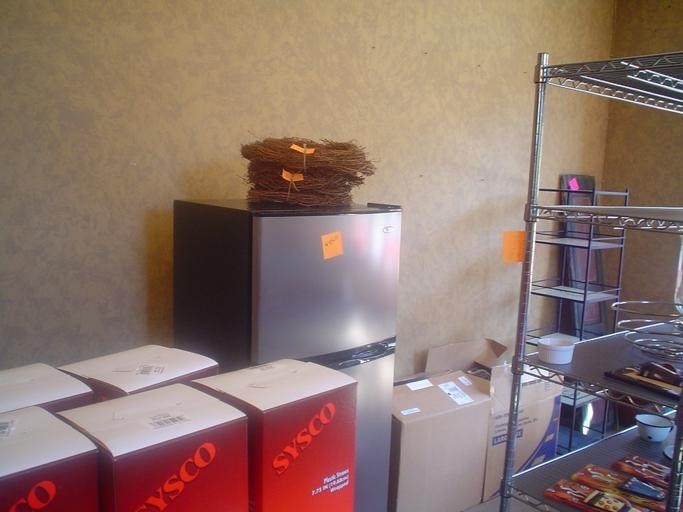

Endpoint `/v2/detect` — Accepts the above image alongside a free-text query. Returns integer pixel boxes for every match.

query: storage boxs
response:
[428,337,558,499]
[56,344,218,395]
[0,403,103,512]
[189,357,359,512]
[0,356,96,410]
[57,383,249,509]
[390,371,492,511]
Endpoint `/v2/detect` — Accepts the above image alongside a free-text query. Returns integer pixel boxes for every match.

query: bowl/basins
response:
[536,337,574,365]
[635,413,672,442]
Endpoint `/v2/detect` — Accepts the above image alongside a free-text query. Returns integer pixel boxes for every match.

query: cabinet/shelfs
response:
[495,47,683,512]
[528,182,630,452]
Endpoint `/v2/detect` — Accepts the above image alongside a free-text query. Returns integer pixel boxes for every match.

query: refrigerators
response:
[172,194,402,509]
